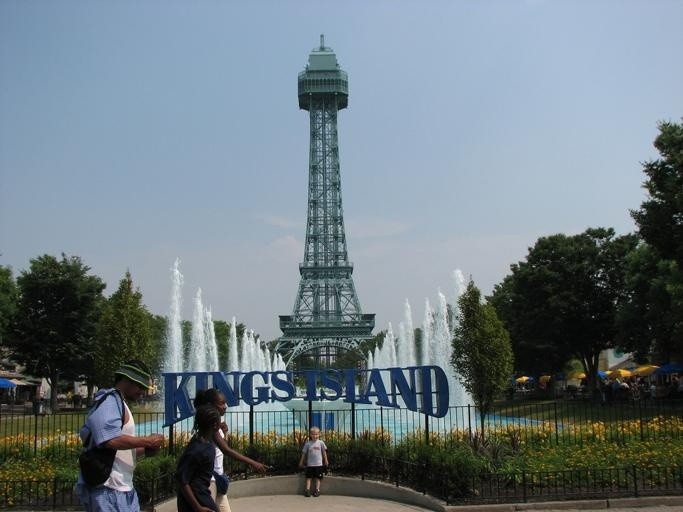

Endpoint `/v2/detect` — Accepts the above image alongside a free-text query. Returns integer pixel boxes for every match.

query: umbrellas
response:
[18,379,38,397]
[9,378,25,399]
[0,377,15,389]
[510,361,682,387]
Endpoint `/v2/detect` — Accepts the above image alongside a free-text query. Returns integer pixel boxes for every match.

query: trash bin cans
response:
[33,401,44,414]
[504,388,513,400]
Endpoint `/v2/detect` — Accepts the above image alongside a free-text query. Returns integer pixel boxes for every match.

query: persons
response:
[193,387,268,511]
[576,374,682,405]
[74,359,162,511]
[297,426,329,496]
[176,405,221,511]
[66,390,71,405]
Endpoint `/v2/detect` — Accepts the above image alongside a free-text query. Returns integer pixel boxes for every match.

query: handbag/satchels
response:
[79,446,117,485]
[215,475,229,495]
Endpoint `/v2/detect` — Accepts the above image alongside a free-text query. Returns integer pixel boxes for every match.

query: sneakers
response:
[304,489,311,496]
[313,489,320,497]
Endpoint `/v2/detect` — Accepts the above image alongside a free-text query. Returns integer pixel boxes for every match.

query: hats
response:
[113,364,152,389]
